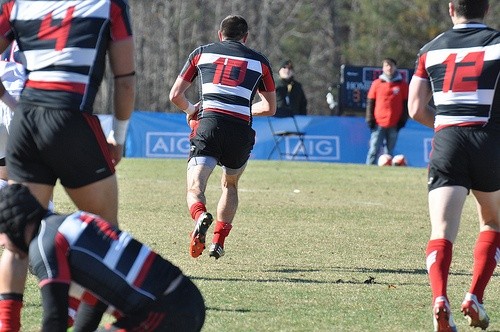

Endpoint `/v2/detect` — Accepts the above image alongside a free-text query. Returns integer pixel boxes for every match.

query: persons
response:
[169,15,276,259]
[274,60,307,118]
[0,0,137,332]
[366,58,409,164]
[0,183,206,332]
[408,0,500,332]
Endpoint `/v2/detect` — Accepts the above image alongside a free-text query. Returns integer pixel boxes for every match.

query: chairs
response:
[265,105,310,163]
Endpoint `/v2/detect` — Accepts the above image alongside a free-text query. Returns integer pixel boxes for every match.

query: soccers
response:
[188,110,199,130]
[378,153,392,167]
[391,154,406,166]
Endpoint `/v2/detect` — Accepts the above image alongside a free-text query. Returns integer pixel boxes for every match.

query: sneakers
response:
[460,293,491,330]
[208,243,226,259]
[430,296,456,332]
[189,212,214,258]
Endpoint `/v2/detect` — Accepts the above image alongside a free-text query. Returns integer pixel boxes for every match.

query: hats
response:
[0,184,42,248]
